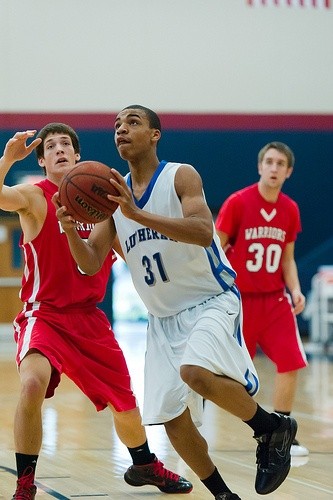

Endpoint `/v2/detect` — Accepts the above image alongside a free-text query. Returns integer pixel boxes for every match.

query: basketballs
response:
[56,160,119,224]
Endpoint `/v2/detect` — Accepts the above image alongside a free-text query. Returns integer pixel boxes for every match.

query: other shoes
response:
[290,439,308,457]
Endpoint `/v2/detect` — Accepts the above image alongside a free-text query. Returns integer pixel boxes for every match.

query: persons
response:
[213,143,310,456]
[51,104,298,500]
[0,123,194,500]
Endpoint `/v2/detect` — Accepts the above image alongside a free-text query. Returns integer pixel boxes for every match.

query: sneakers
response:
[252,412,298,495]
[11,466,37,500]
[124,453,193,494]
[214,491,241,500]
